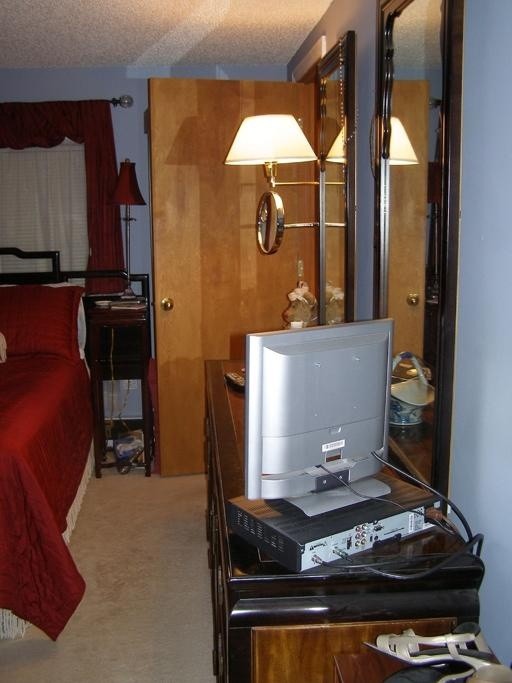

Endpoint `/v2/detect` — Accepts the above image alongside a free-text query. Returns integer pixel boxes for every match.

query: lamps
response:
[109,157,146,302]
[224,111,317,186]
[326,115,419,164]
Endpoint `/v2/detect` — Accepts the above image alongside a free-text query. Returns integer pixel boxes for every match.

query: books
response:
[115,428,146,468]
[112,303,148,310]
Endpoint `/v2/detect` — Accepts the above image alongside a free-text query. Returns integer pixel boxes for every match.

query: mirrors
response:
[374,2,462,492]
[313,30,356,326]
[255,190,285,256]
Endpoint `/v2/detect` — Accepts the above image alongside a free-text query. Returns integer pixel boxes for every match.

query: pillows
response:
[0,284,85,362]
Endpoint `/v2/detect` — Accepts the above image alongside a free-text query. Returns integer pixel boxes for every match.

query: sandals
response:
[361,622,500,683]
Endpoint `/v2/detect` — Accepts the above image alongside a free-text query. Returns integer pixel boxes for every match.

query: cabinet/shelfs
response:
[204,359,499,682]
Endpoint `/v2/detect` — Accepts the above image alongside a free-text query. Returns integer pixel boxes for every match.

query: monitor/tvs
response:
[246,317,396,516]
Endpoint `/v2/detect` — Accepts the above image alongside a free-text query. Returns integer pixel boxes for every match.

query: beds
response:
[0,245,94,645]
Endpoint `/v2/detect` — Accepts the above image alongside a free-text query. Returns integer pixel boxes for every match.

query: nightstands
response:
[82,276,155,478]
